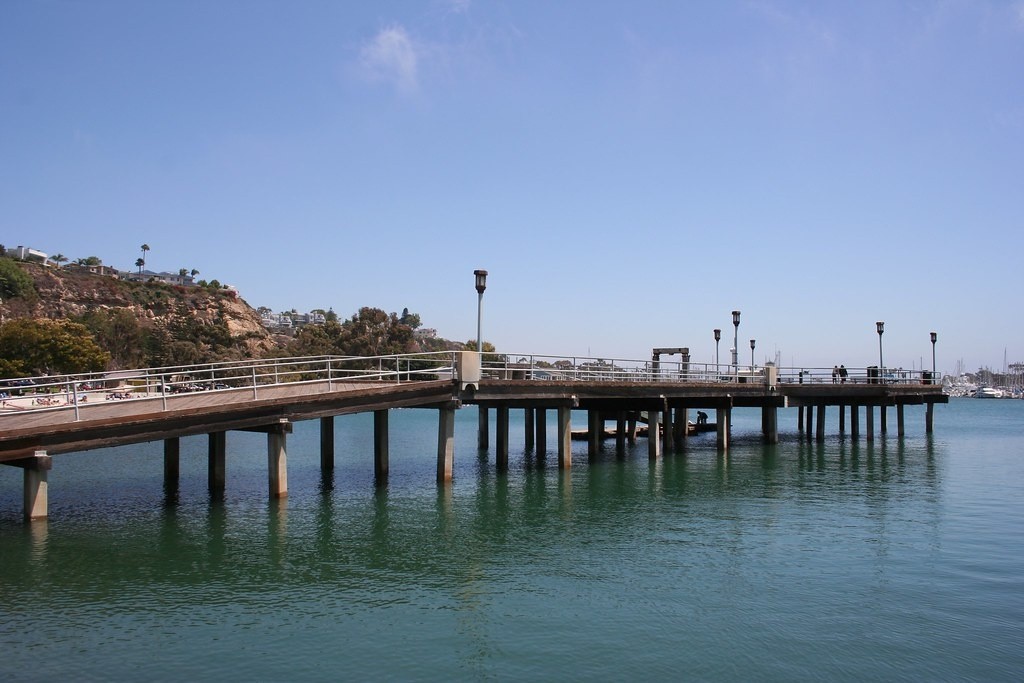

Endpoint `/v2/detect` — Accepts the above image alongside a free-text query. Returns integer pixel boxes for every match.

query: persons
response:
[80,396,87,402]
[832,365,848,384]
[697,411,708,424]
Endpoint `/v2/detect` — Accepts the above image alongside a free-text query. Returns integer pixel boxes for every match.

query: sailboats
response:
[941,357,1024,399]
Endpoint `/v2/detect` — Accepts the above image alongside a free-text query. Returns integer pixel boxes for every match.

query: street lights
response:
[930,332,938,386]
[876,321,885,385]
[750,339,756,384]
[732,310,741,382]
[714,329,721,383]
[474,269,488,379]
